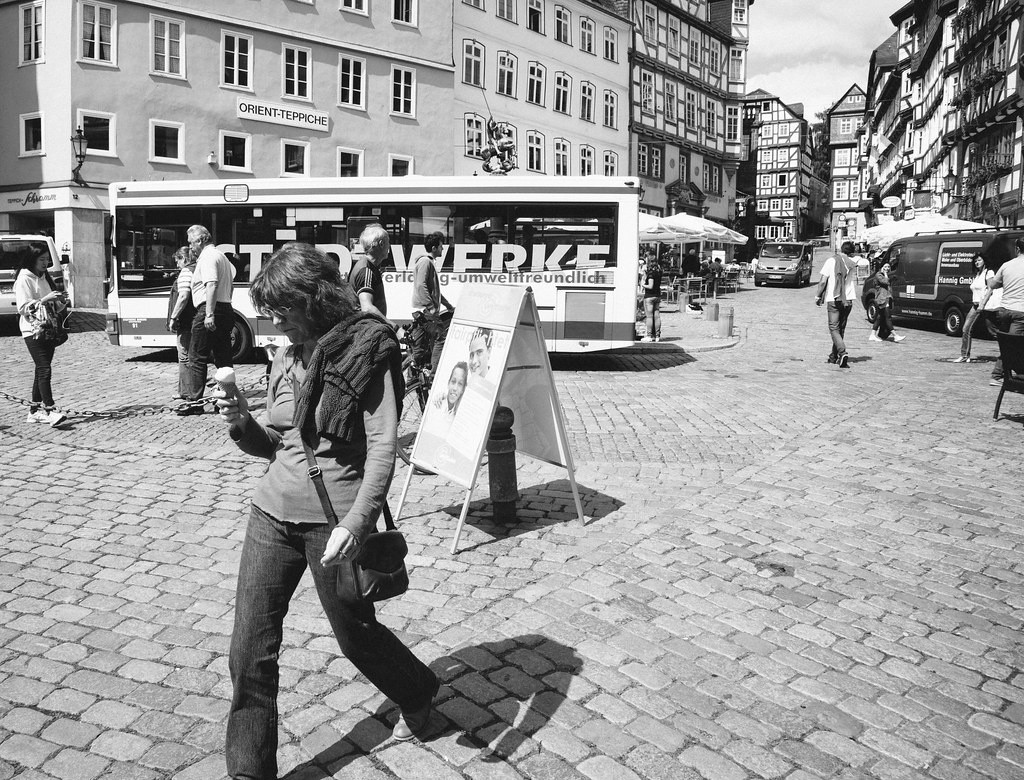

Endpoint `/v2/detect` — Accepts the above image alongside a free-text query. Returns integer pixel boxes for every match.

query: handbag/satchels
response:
[29,300,70,348]
[336,528,410,604]
[979,287,1004,312]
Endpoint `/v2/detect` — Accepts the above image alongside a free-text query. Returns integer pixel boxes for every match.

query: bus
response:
[103,174,646,365]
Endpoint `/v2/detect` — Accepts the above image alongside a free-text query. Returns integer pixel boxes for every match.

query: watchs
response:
[206,313,213,318]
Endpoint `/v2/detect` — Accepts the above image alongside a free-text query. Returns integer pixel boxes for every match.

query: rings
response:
[340,551,349,560]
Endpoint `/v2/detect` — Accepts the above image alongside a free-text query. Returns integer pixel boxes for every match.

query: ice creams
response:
[215,366,236,400]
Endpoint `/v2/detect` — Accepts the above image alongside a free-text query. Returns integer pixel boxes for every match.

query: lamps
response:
[899,174,921,189]
[639,186,646,201]
[942,168,968,196]
[70,125,89,174]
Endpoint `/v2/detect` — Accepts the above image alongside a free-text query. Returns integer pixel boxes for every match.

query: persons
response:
[814,242,857,368]
[407,231,453,387]
[347,223,407,341]
[641,263,661,343]
[698,256,722,292]
[988,238,1024,386]
[953,253,1002,363]
[14,242,67,427]
[165,224,234,415]
[866,250,876,263]
[681,249,701,277]
[213,242,440,780]
[433,327,496,421]
[868,262,906,343]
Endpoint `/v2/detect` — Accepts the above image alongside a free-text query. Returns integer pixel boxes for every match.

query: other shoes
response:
[174,406,205,416]
[171,394,182,399]
[840,354,848,368]
[828,359,834,364]
[641,337,659,342]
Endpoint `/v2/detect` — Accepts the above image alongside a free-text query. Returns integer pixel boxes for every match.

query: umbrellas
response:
[639,212,749,265]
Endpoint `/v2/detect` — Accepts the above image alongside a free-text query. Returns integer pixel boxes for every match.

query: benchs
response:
[989,332,1024,420]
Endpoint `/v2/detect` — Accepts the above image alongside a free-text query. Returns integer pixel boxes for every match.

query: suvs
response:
[0,229,70,329]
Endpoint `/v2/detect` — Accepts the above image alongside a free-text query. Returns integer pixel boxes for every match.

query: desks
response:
[674,277,701,303]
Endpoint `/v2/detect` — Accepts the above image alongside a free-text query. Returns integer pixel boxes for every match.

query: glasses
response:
[261,306,297,318]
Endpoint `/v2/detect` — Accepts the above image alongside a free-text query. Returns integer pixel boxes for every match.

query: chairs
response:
[658,269,741,303]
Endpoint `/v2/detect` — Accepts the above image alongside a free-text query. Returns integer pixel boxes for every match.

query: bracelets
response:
[394,324,399,333]
[815,296,821,299]
[170,315,177,320]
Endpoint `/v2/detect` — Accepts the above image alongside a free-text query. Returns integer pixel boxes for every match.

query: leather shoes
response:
[393,676,441,741]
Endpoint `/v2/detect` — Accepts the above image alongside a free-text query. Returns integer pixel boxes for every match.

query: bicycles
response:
[390,309,453,474]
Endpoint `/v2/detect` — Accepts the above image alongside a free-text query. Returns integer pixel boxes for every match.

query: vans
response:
[861,224,1024,337]
[754,241,813,288]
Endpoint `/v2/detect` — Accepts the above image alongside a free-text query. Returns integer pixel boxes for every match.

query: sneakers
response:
[953,357,971,363]
[894,335,906,342]
[989,378,1004,387]
[27,410,67,427]
[869,335,882,342]
